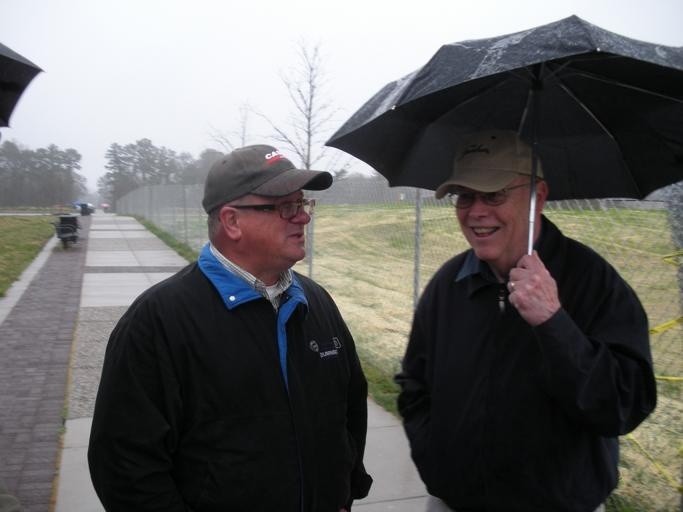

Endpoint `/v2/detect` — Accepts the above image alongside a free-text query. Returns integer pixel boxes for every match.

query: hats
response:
[202,144,333,214]
[435,131,545,201]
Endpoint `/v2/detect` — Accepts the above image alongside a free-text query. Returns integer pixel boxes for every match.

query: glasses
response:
[446,179,540,211]
[218,197,317,225]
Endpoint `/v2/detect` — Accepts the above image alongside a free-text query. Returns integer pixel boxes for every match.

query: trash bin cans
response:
[59,215,77,242]
[81,204,88,216]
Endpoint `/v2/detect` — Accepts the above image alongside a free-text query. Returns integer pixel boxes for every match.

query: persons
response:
[87,144,373,511]
[390,130,655,511]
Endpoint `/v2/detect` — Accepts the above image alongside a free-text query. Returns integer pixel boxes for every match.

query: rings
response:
[509,279,516,291]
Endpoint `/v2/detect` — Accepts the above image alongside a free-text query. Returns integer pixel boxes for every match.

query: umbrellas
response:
[322,15,682,255]
[0,43,45,127]
